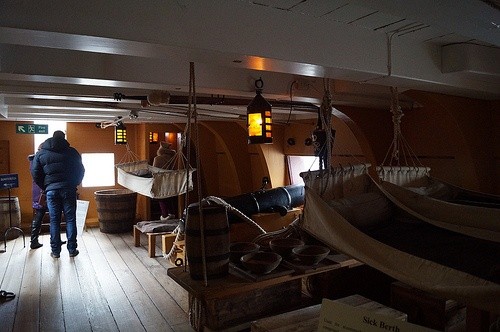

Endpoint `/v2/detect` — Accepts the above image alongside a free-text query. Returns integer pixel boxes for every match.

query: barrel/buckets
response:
[0,196,22,240]
[185,205,231,280]
[95,189,137,232]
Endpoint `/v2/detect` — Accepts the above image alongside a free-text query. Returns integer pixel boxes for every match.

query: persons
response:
[153,141,178,220]
[31,131,85,258]
[28,143,49,249]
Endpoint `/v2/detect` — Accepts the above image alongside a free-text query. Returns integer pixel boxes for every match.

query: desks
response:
[251,293,444,332]
[167,246,358,332]
[133,225,174,257]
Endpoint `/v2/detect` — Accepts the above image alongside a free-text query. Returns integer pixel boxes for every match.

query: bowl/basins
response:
[269,238,305,255]
[240,251,282,274]
[230,241,260,259]
[292,245,331,264]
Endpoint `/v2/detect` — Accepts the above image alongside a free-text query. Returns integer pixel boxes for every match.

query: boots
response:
[31,221,43,249]
[61,240,66,244]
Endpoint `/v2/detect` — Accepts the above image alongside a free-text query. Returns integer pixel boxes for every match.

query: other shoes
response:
[51,253,60,258]
[70,250,79,257]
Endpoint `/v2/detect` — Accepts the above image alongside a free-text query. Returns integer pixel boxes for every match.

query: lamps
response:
[114,116,127,144]
[149,129,159,144]
[246,77,274,145]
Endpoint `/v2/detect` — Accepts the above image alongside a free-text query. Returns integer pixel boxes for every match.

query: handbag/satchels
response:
[39,193,47,207]
